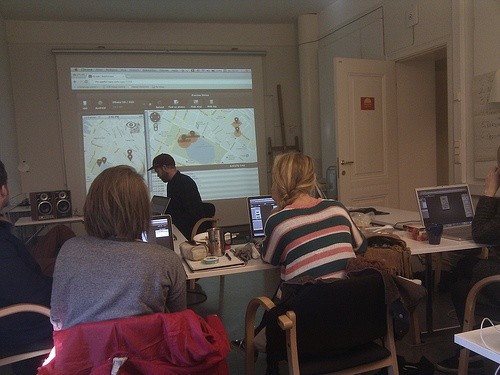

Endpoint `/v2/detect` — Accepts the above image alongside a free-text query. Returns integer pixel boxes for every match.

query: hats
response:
[147,153,174,171]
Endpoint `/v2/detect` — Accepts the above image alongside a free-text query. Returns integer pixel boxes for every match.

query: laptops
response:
[247,196,278,244]
[415,184,475,241]
[140,214,174,253]
[150,194,172,215]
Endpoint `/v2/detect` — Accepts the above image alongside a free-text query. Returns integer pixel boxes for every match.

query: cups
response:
[207,227,225,257]
[425,223,443,245]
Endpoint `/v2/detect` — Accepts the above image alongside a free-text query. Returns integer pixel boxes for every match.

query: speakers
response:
[30,190,72,220]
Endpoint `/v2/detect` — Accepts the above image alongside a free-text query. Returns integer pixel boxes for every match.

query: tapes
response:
[203,256,219,264]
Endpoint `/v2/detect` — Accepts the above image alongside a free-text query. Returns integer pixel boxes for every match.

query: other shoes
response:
[436,355,483,374]
[231,339,259,361]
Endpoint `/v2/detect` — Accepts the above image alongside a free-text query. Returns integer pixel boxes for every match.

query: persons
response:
[50,164,187,331]
[147,154,204,240]
[437,146,500,373]
[230,151,368,362]
[0,161,53,375]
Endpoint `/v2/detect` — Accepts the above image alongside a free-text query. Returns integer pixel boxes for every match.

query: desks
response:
[8,205,500,375]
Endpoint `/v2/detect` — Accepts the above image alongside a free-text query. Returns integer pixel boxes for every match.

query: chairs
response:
[245,265,398,375]
[0,303,52,375]
[456,272,500,375]
[189,202,217,289]
[34,307,231,375]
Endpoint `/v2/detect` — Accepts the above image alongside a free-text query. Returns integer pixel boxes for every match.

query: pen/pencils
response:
[226,252,231,261]
